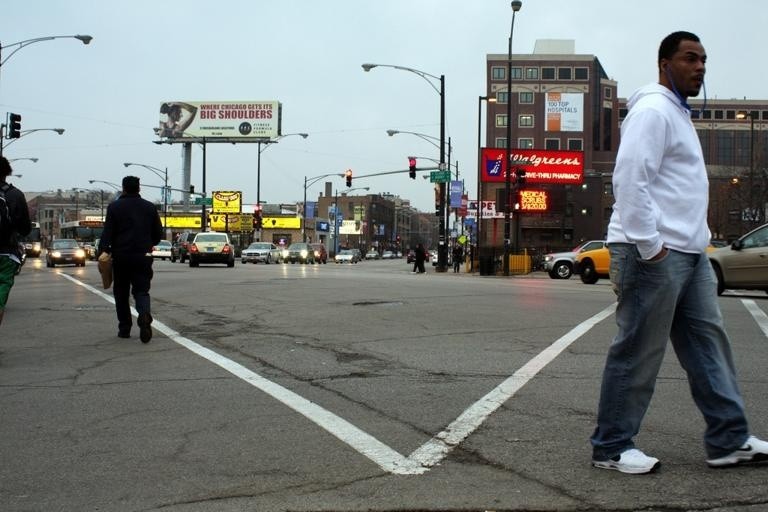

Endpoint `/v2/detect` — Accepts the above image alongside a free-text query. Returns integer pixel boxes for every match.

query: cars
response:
[240,241,281,264]
[705,223,768,295]
[334,250,357,263]
[77,239,101,261]
[151,239,173,262]
[44,238,85,268]
[573,243,728,285]
[365,249,452,267]
[188,233,236,266]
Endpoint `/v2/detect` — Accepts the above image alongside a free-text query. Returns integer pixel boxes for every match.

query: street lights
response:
[476,95,502,255]
[502,0,522,275]
[303,172,345,243]
[736,111,754,222]
[1,129,66,180]
[123,162,169,239]
[728,174,745,232]
[360,63,448,272]
[386,128,451,244]
[88,177,125,195]
[422,173,430,180]
[334,187,371,256]
[0,35,93,69]
[256,132,310,207]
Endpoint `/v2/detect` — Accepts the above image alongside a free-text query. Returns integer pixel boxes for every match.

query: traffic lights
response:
[252,210,262,229]
[512,189,522,212]
[207,218,210,226]
[191,186,194,194]
[8,112,22,139]
[344,168,352,187]
[516,169,526,190]
[408,154,417,179]
[396,235,401,246]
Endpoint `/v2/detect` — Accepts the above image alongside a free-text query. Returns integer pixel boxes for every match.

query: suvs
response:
[350,248,362,260]
[310,242,327,264]
[170,231,197,262]
[540,240,607,279]
[284,241,313,264]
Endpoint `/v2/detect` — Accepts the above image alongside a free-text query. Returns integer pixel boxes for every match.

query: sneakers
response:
[591,449,660,475]
[706,435,768,470]
[139,314,152,344]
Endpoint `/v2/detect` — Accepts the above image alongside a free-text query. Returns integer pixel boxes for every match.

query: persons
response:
[0,157,32,325]
[590,31,768,474]
[453,245,463,273]
[418,244,425,273]
[98,176,162,342]
[158,102,197,138]
[412,244,421,273]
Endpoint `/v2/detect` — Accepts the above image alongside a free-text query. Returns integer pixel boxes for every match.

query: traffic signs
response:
[428,169,453,183]
[195,198,212,205]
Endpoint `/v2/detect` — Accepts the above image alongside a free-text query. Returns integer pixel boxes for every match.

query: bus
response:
[21,221,41,258]
[60,220,106,257]
